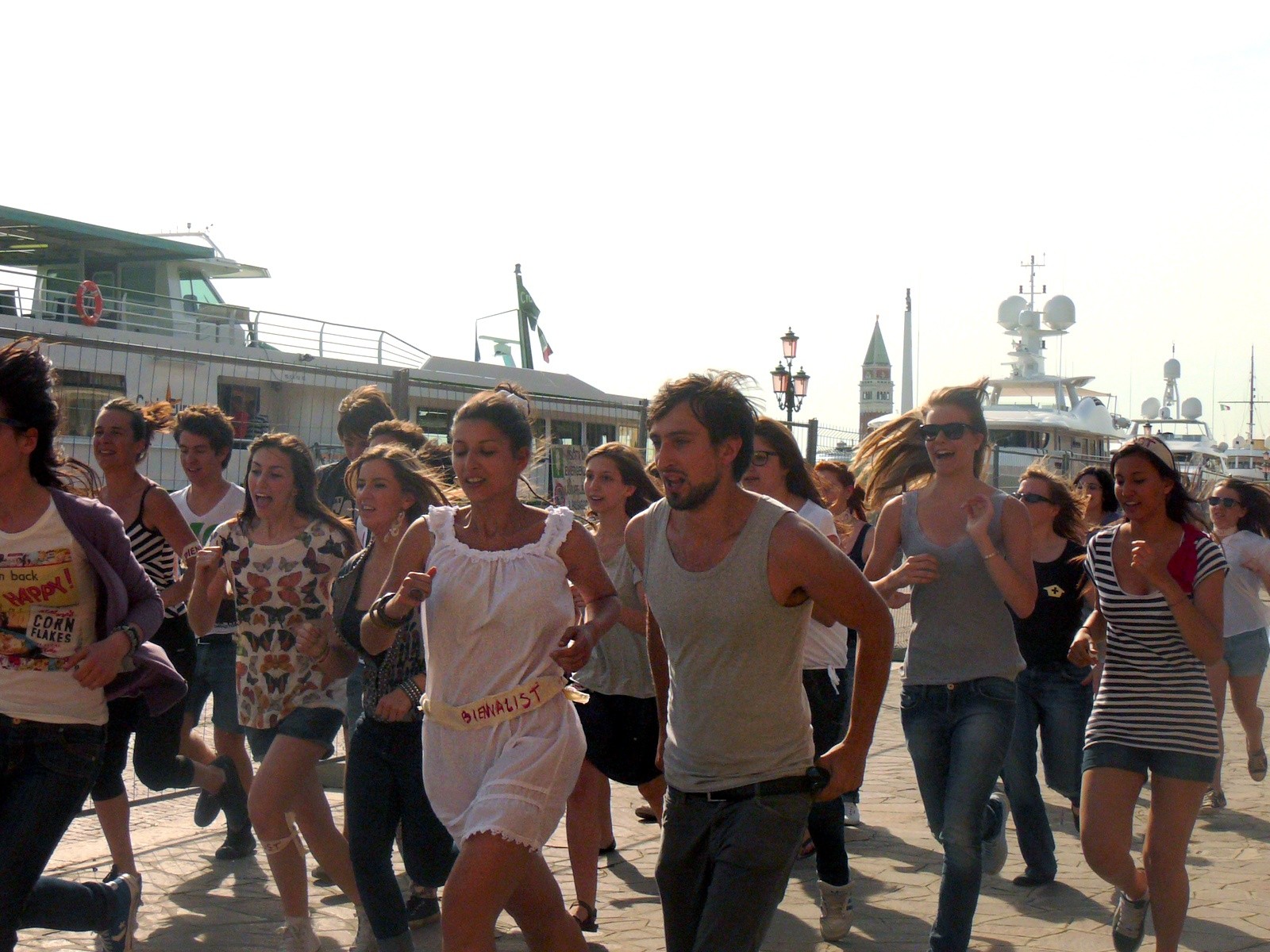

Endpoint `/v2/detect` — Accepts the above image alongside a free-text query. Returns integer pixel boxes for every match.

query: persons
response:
[157,403,264,862]
[341,418,446,934]
[357,383,623,952]
[620,371,897,952]
[596,459,664,856]
[565,442,675,934]
[329,442,454,951]
[186,429,371,951]
[737,416,857,946]
[0,333,190,952]
[798,826,819,859]
[808,460,883,828]
[81,395,237,911]
[1070,465,1124,703]
[999,450,1106,889]
[1064,435,1232,951]
[852,379,1041,952]
[1198,474,1270,814]
[302,381,410,882]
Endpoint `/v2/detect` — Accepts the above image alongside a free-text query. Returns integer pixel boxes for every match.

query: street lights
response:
[769,326,811,444]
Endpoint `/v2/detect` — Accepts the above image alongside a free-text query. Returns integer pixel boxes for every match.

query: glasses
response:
[919,421,980,441]
[749,450,780,466]
[1207,497,1243,507]
[1074,482,1105,491]
[1011,491,1054,505]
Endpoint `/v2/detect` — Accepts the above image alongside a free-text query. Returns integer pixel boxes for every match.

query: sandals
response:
[795,828,818,860]
[567,901,597,927]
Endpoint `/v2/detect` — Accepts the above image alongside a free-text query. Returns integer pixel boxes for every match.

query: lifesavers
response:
[77,281,103,324]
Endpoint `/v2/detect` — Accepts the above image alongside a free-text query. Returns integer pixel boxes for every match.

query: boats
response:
[1211,344,1270,488]
[867,253,1131,496]
[0,207,654,532]
[1110,344,1232,502]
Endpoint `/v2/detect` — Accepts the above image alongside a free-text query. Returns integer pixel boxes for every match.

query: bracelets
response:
[367,598,400,630]
[376,592,414,628]
[110,620,141,657]
[1168,596,1190,610]
[981,548,1001,561]
[398,678,424,709]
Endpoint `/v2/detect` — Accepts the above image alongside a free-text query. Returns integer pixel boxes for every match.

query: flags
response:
[516,282,553,363]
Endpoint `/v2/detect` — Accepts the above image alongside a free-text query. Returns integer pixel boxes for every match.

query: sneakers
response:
[216,825,256,860]
[981,792,1011,876]
[1246,736,1267,782]
[634,806,657,823]
[96,873,134,952]
[349,905,379,952]
[312,864,326,879]
[1014,871,1052,884]
[404,895,441,924]
[1112,867,1149,951]
[194,786,222,827]
[208,756,248,824]
[599,838,617,856]
[279,917,322,952]
[1196,787,1227,814]
[843,801,861,825]
[818,879,857,942]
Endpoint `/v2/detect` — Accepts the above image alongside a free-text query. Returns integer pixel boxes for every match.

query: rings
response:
[408,587,426,603]
